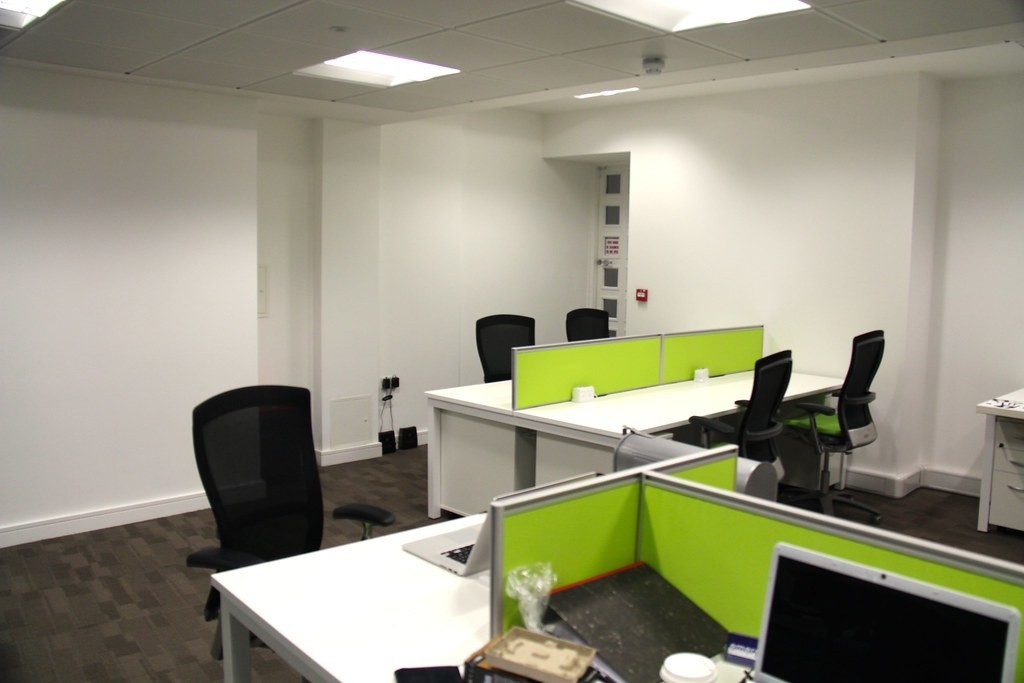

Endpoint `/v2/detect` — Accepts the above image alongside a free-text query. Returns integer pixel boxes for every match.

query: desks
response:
[424,325,846,521]
[976,387,1024,533]
[209,442,759,683]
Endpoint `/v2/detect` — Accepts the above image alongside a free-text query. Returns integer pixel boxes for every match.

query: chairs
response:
[679,349,806,466]
[184,384,397,657]
[565,309,610,343]
[476,315,537,382]
[782,329,887,524]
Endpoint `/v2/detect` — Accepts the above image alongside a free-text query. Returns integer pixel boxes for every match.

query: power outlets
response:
[381,384,397,401]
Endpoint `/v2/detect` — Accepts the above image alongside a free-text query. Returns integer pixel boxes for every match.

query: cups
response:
[659,652,719,683]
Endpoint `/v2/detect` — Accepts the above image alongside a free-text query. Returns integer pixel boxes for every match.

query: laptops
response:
[752,543,1020,683]
[403,472,597,577]
